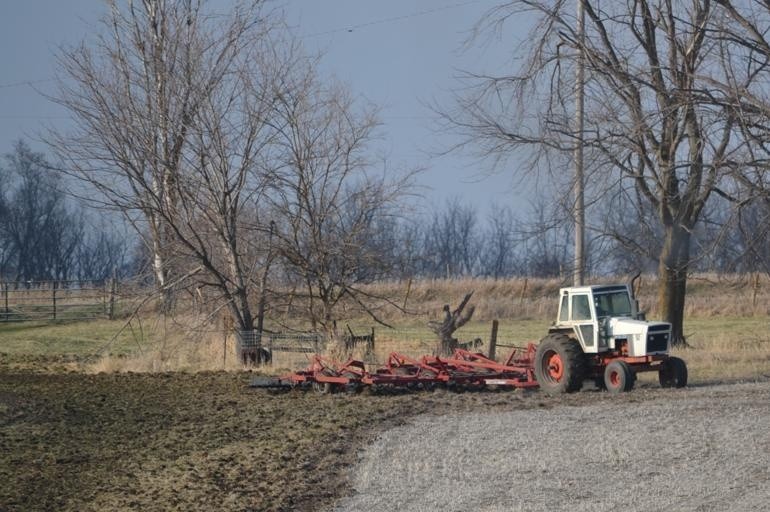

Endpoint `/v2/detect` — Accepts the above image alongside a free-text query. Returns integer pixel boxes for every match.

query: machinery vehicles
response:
[533,281,689,398]
